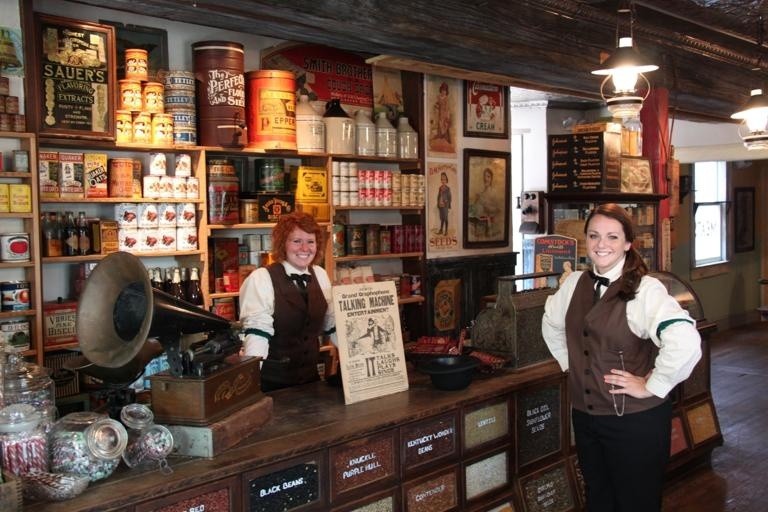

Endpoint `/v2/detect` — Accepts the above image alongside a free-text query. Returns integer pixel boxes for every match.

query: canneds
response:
[158,227,177,252]
[138,228,159,251]
[391,190,402,206]
[399,272,411,299]
[0,114,11,133]
[131,111,152,145]
[418,175,425,191]
[174,153,192,177]
[390,276,400,296]
[141,80,165,115]
[340,162,348,176]
[399,174,410,192]
[176,202,196,227]
[176,227,198,251]
[391,172,401,191]
[132,178,142,199]
[332,191,339,206]
[149,152,168,176]
[0,95,5,114]
[383,187,393,207]
[114,110,133,143]
[383,171,392,189]
[358,169,365,188]
[186,177,199,199]
[350,192,358,206]
[358,188,365,207]
[365,187,375,207]
[156,202,177,226]
[409,191,418,206]
[331,161,340,176]
[12,115,26,132]
[150,113,174,146]
[411,274,420,295]
[348,176,359,192]
[116,79,142,113]
[0,280,31,312]
[112,203,137,228]
[366,169,375,188]
[11,149,29,173]
[0,232,31,264]
[143,175,160,197]
[375,170,384,189]
[331,175,340,191]
[123,48,149,82]
[340,191,349,205]
[0,319,32,353]
[417,192,425,206]
[374,187,383,207]
[132,159,142,180]
[401,190,410,206]
[4,96,19,116]
[137,202,159,229]
[339,176,349,191]
[0,76,10,96]
[117,227,138,252]
[348,162,359,176]
[173,176,186,198]
[159,175,174,198]
[409,174,418,191]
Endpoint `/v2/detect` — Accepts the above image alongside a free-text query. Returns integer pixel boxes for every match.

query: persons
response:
[435,171,451,236]
[541,204,704,512]
[239,213,337,393]
[468,168,502,241]
[433,82,452,144]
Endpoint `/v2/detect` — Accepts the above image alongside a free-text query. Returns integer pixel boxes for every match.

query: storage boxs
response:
[547,131,622,192]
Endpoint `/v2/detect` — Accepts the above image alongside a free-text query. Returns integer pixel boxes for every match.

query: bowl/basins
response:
[417,354,480,389]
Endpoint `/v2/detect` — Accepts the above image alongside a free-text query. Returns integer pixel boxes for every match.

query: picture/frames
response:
[734,186,755,252]
[619,157,656,193]
[463,80,508,139]
[31,11,117,142]
[462,148,511,249]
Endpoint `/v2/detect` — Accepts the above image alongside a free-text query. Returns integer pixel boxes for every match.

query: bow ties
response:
[587,269,611,305]
[289,273,312,290]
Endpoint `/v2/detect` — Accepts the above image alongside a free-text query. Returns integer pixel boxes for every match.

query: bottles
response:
[148,267,205,311]
[123,403,174,475]
[376,112,397,156]
[40,209,93,257]
[397,117,420,158]
[296,95,325,153]
[50,412,129,485]
[0,403,50,474]
[322,98,356,154]
[207,160,239,225]
[0,355,54,477]
[356,109,376,155]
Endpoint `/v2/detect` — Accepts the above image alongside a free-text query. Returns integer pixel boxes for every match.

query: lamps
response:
[591,0,659,118]
[730,16,768,152]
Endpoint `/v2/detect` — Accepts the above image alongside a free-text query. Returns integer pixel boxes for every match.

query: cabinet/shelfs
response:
[0,130,44,367]
[36,138,428,405]
